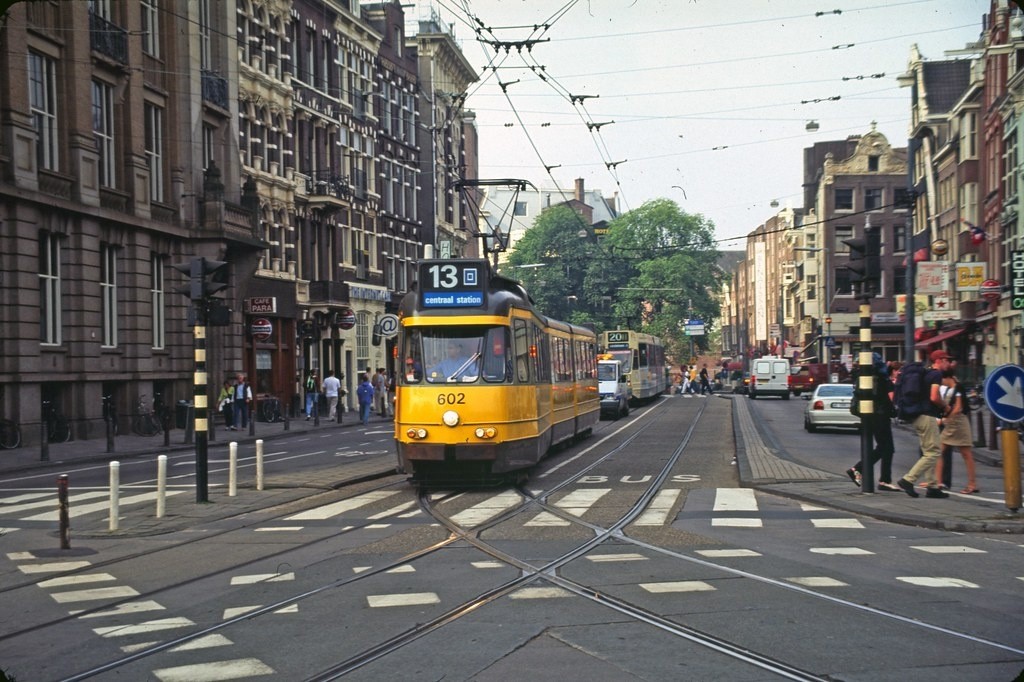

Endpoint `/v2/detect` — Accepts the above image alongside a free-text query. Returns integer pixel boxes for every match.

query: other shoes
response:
[846,467,862,487]
[305,415,311,421]
[925,487,950,498]
[960,488,979,494]
[231,426,237,431]
[878,481,900,492]
[898,478,919,498]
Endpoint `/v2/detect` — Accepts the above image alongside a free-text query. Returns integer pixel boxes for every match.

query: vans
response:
[790,363,848,396]
[598,360,632,420]
[748,356,792,400]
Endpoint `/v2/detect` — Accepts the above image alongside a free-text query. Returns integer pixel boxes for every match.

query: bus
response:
[393,258,599,490]
[599,331,667,401]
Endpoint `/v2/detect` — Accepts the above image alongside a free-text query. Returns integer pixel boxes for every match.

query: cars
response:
[801,384,861,433]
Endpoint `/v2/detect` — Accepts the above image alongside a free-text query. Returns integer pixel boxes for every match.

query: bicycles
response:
[101,395,118,433]
[134,392,166,437]
[967,384,983,410]
[262,394,282,423]
[0,418,21,449]
[43,401,70,444]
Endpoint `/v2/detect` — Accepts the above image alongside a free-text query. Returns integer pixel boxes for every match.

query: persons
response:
[846,361,905,489]
[321,370,340,421]
[897,350,951,500]
[366,367,396,419]
[919,353,959,491]
[216,381,234,430]
[230,373,253,430]
[424,340,486,382]
[700,363,715,395]
[601,366,615,380]
[680,367,694,394]
[356,376,373,423]
[302,369,321,420]
[687,366,700,395]
[919,370,980,494]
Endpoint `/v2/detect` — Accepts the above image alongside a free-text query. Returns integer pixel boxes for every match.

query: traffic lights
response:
[172,258,191,298]
[202,257,228,300]
[841,226,882,280]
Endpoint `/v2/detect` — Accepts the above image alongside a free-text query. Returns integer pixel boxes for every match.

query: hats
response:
[929,349,955,361]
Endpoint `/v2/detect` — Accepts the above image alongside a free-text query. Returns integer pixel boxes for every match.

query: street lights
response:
[793,247,832,384]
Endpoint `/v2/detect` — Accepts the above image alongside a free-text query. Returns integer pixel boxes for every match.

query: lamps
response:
[806,120,819,131]
[770,200,779,208]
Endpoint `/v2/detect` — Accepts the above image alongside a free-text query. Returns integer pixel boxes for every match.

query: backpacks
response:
[305,376,315,391]
[894,362,939,424]
